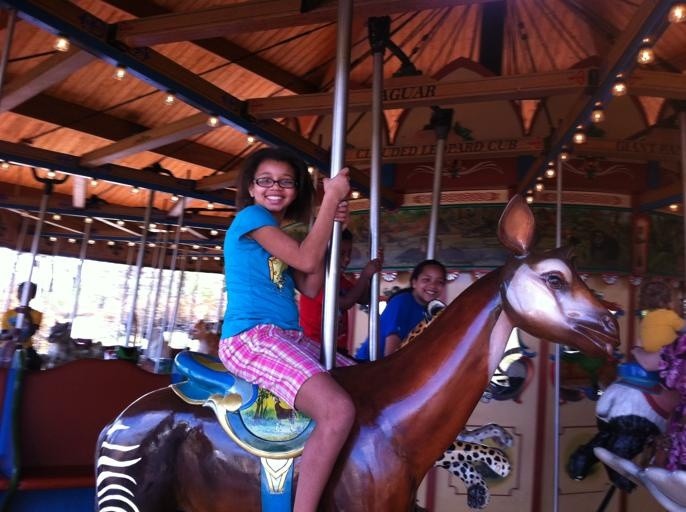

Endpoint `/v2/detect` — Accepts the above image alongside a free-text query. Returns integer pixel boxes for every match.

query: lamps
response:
[1,159,220,237]
[525,1,686,210]
[51,28,258,146]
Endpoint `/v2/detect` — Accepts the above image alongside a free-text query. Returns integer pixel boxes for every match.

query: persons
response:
[218,147,357,512]
[353,260,447,362]
[0,281,44,371]
[299,227,385,358]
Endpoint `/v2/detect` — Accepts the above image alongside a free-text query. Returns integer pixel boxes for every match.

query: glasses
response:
[254,177,297,189]
